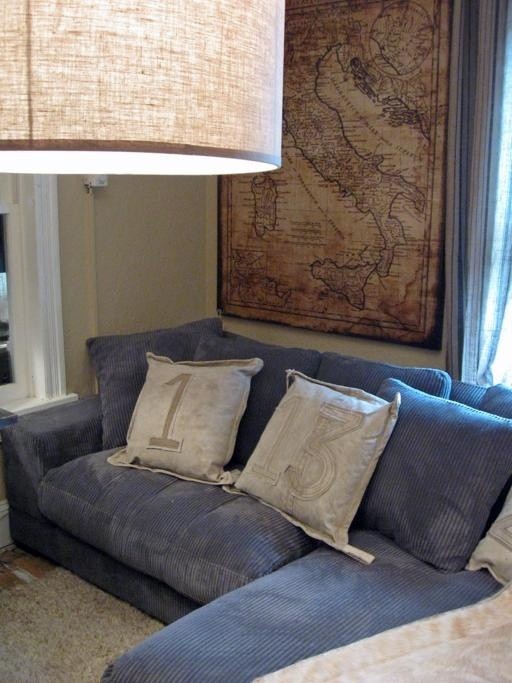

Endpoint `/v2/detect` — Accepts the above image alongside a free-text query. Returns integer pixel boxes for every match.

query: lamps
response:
[0,0,284,181]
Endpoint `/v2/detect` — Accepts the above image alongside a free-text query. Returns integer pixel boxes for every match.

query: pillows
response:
[318,349,453,408]
[193,329,321,467]
[478,382,511,419]
[106,348,264,492]
[221,368,402,573]
[84,316,226,456]
[465,484,511,584]
[355,375,512,577]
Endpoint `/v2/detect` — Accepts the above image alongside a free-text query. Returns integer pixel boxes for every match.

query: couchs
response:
[0,330,512,681]
